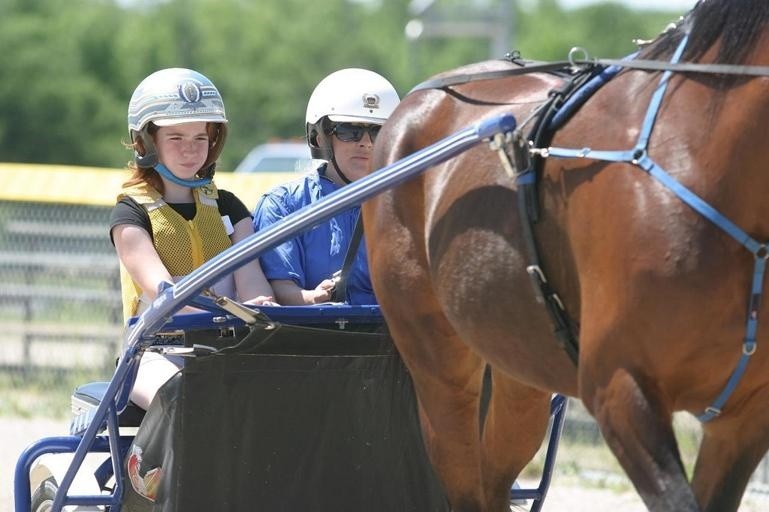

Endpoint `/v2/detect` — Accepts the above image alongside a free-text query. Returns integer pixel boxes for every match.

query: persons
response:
[104,65,281,413]
[251,67,403,305]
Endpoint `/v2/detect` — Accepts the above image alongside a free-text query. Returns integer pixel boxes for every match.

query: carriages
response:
[9,1,767,511]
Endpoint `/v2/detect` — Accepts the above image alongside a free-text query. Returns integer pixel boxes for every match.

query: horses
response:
[360,0,768,512]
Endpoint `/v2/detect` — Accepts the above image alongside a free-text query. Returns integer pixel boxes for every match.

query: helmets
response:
[127,68,229,168]
[305,68,400,159]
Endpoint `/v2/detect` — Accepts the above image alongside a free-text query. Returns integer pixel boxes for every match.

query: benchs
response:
[70,380,147,434]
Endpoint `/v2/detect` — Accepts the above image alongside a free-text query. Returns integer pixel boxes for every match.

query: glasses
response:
[328,124,381,144]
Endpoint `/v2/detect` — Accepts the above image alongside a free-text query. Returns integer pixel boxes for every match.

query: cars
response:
[231,140,323,174]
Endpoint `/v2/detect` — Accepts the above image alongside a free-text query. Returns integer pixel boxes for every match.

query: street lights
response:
[402,14,510,59]
